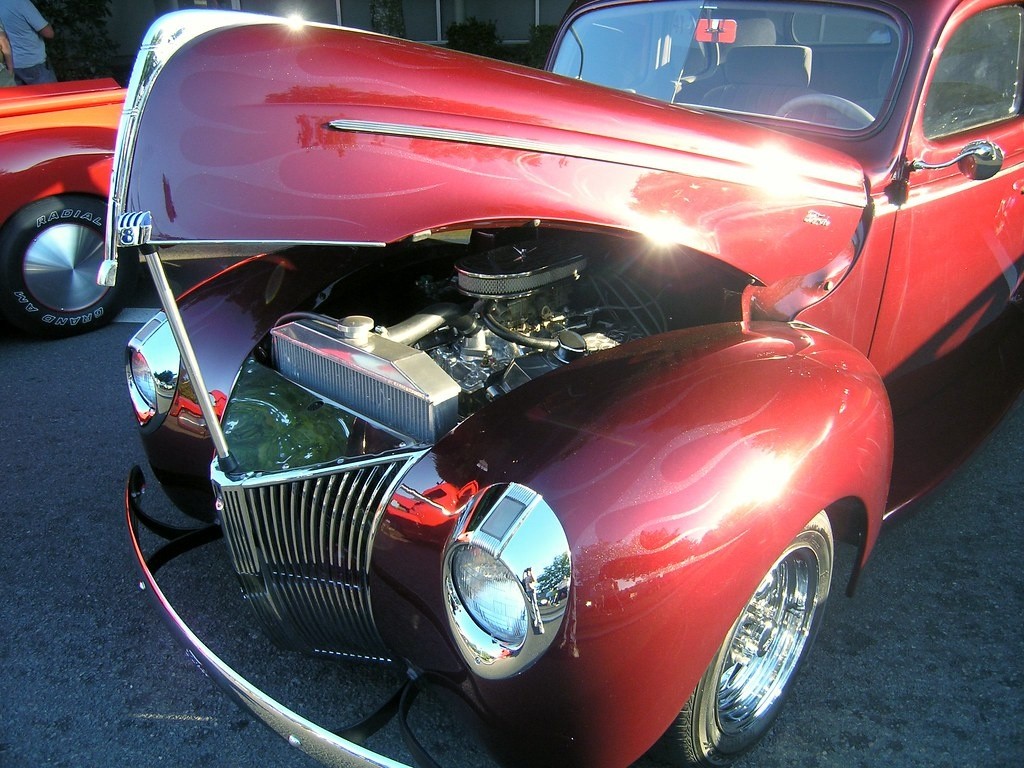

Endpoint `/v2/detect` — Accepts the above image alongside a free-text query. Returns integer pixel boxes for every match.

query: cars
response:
[1,75,136,338]
[96,0,1024,764]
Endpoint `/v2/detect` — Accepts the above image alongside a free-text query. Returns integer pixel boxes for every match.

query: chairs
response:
[699,42,825,127]
[845,58,896,128]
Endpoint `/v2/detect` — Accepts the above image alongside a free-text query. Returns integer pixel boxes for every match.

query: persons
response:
[0,0,57,86]
[0,22,14,87]
